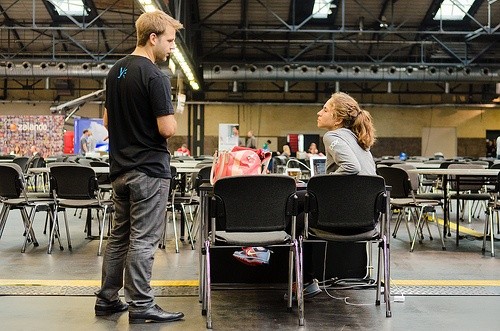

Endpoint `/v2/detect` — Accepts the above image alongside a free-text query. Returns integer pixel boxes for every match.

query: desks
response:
[418,166,500,238]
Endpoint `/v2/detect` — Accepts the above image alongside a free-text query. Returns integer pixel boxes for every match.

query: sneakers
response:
[234,246,271,266]
[283,279,321,302]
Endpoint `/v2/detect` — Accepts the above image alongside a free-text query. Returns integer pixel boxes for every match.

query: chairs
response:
[158,167,211,253]
[0,154,115,254]
[294,175,392,317]
[375,158,500,257]
[200,175,304,329]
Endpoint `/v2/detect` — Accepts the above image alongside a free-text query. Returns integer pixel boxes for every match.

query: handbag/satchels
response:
[210,146,273,184]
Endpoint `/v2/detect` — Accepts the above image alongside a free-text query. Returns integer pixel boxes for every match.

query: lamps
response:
[5,62,108,71]
[208,65,491,76]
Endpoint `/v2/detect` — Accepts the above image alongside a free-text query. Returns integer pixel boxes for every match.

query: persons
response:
[247,130,256,149]
[263,139,272,151]
[284,91,377,302]
[95,11,184,323]
[308,143,318,154]
[266,145,291,173]
[177,144,190,157]
[86,131,95,153]
[486,136,500,159]
[80,129,89,156]
[220,126,239,144]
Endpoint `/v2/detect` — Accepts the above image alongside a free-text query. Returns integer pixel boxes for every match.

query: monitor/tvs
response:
[310,156,326,176]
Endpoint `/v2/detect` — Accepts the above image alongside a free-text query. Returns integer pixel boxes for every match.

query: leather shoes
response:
[94,302,129,315]
[129,303,185,324]
